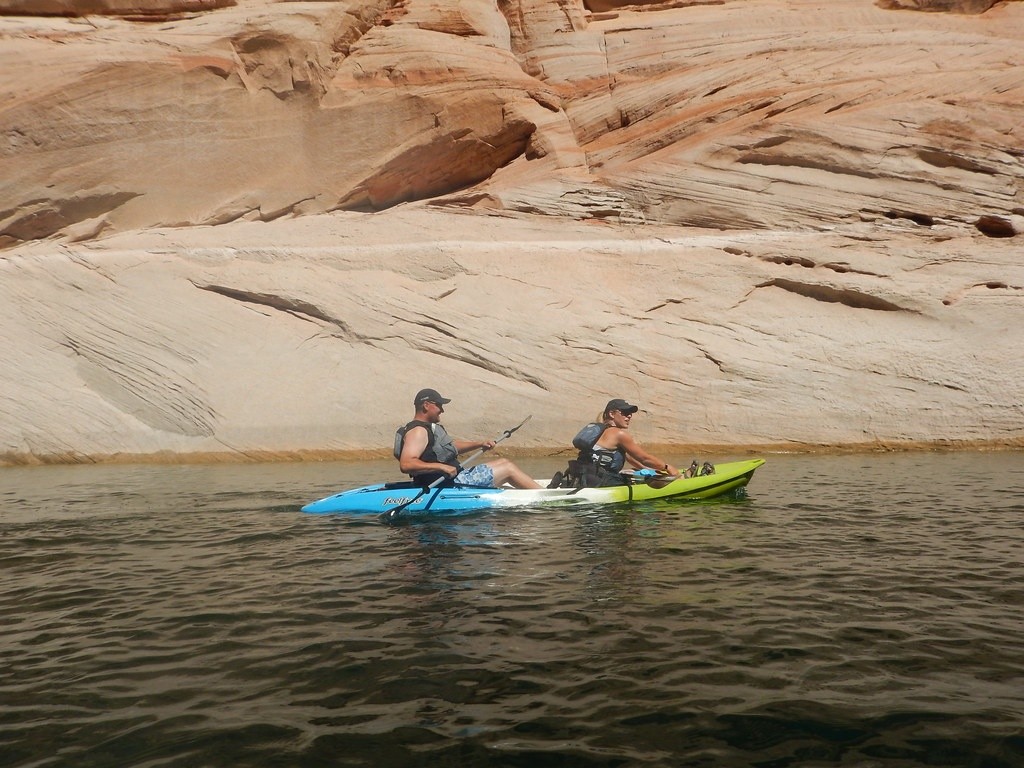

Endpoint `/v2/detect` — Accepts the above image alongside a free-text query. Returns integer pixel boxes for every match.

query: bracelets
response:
[664,463,669,471]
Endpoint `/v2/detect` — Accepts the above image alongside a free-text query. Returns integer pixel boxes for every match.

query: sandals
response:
[700,461,715,475]
[683,459,699,478]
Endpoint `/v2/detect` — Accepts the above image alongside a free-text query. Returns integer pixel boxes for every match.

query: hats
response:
[414,388,451,404]
[605,399,638,413]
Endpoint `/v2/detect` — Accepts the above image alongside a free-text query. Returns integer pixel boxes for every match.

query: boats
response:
[300,458,768,517]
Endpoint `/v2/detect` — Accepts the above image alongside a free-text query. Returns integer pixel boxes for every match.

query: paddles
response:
[374,413,533,525]
[621,472,678,489]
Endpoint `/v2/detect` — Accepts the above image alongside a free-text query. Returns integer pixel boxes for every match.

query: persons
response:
[399,389,563,489]
[578,399,716,485]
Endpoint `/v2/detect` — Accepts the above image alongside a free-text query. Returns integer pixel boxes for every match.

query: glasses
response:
[613,410,634,416]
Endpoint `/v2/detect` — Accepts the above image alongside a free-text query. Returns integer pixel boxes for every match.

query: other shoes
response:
[547,470,563,488]
